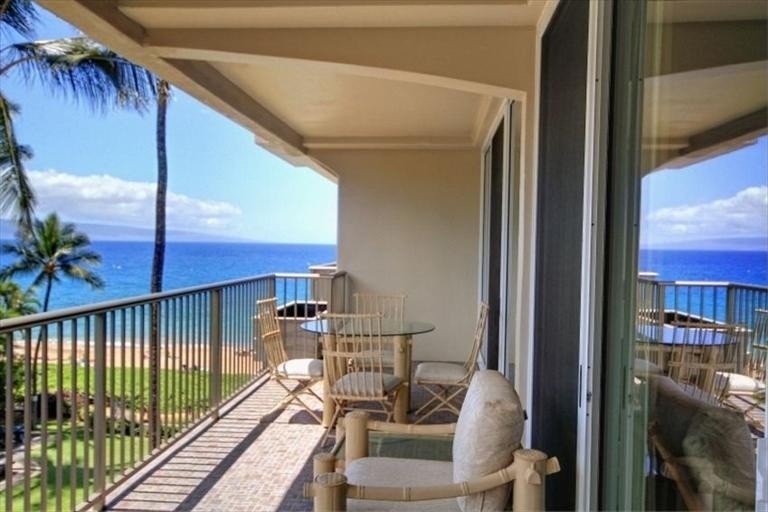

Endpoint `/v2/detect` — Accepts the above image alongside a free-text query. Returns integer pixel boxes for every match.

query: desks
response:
[298,314,438,434]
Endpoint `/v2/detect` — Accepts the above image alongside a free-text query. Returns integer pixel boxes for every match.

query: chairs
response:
[635,303,768,512]
[302,368,563,512]
[409,297,496,424]
[307,305,404,452]
[245,295,325,434]
[347,293,411,376]
